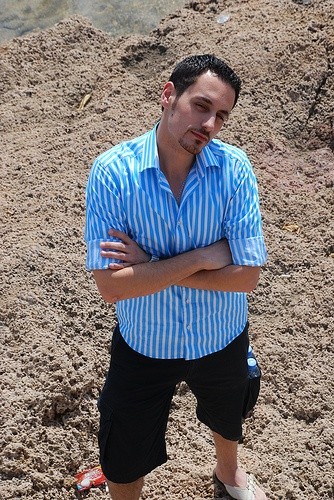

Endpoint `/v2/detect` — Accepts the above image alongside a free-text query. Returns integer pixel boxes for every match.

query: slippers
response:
[212,462,268,500]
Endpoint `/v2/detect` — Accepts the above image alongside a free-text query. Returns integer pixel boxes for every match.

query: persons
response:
[84,54,272,500]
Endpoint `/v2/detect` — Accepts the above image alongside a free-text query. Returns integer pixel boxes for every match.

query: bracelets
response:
[148,254,159,263]
